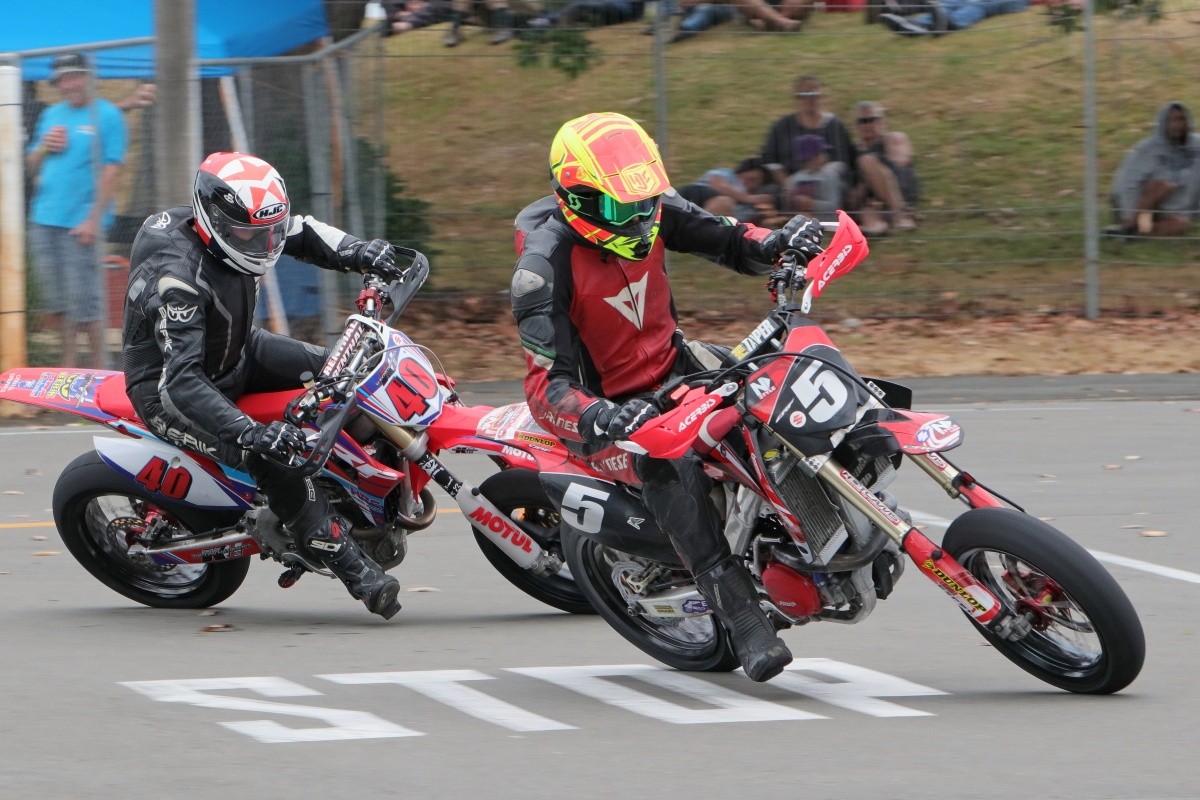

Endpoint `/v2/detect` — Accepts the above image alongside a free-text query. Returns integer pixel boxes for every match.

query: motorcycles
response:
[0,244,598,615]
[475,208,1147,695]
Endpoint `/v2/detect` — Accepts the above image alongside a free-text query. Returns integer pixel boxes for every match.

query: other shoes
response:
[442,31,464,47]
[892,212,916,231]
[879,12,928,38]
[488,27,517,46]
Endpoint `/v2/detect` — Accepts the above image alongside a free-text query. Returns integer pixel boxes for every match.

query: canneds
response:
[51,126,68,152]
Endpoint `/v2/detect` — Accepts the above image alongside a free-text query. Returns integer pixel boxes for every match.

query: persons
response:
[510,111,827,682]
[19,53,128,367]
[378,0,811,47]
[878,0,1085,38]
[672,77,920,237]
[1098,102,1200,243]
[122,152,403,620]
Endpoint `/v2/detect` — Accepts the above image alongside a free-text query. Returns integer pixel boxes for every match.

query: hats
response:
[856,101,883,119]
[792,134,832,161]
[791,76,824,96]
[49,52,93,79]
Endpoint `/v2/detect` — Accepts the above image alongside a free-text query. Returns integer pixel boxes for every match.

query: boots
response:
[697,554,793,681]
[285,482,402,620]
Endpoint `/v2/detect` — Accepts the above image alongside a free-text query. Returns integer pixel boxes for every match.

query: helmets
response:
[549,112,671,261]
[192,151,289,277]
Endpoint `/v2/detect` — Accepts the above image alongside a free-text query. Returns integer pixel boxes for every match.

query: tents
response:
[0,0,365,339]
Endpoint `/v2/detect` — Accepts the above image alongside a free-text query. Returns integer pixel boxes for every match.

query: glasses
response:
[598,192,655,226]
[858,118,875,125]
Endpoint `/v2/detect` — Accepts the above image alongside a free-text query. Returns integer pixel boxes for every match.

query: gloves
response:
[761,214,825,255]
[594,399,662,444]
[252,420,307,467]
[337,234,404,278]
